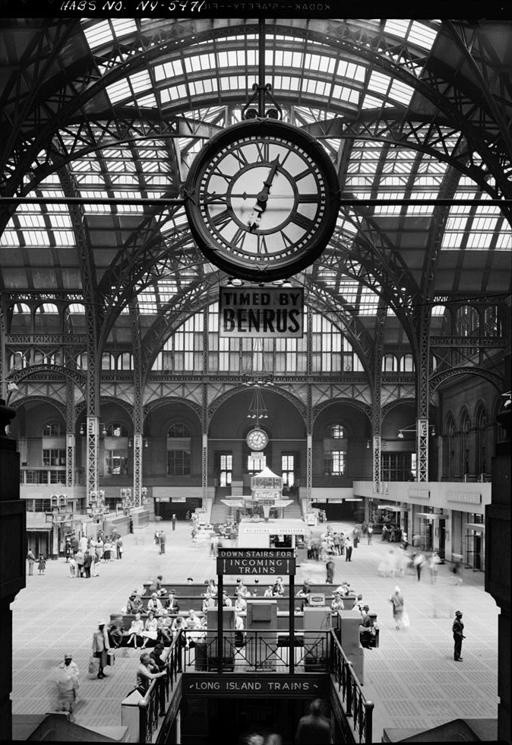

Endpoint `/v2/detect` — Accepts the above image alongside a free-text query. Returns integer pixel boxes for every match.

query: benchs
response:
[106,585,355,647]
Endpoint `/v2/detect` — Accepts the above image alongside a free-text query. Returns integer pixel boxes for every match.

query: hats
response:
[98,622,105,626]
[65,654,72,659]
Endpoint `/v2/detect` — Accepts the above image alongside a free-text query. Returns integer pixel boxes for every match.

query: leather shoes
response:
[97,671,106,679]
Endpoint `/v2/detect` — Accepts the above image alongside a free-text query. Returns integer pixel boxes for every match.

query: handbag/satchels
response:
[89,657,100,674]
[107,653,115,666]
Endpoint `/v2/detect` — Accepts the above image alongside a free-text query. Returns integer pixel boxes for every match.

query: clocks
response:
[245,428,269,451]
[181,116,342,284]
